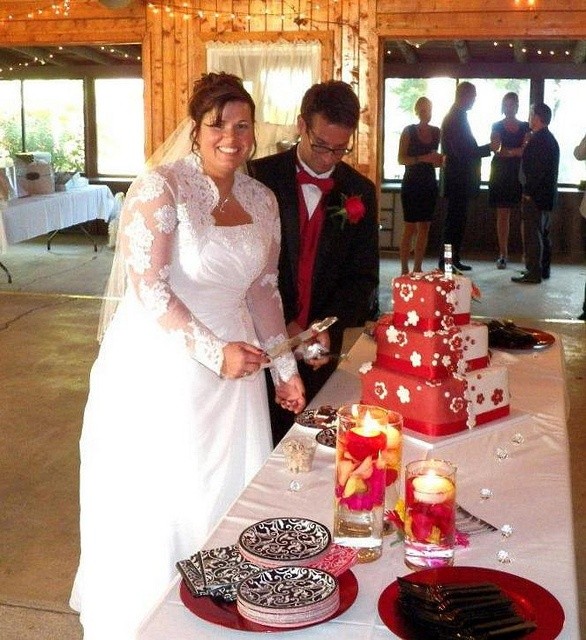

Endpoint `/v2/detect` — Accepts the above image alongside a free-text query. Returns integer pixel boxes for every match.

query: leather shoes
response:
[521,269,549,279]
[512,274,541,284]
[438,263,462,275]
[452,262,471,271]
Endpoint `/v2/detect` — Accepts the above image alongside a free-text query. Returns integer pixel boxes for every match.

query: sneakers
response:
[497,258,507,269]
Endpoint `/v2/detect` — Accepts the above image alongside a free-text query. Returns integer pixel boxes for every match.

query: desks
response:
[0,185,115,284]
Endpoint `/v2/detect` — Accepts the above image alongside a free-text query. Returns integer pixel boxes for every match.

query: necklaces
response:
[217,194,233,213]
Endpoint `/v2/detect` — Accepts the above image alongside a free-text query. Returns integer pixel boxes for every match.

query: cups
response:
[332,403,389,563]
[382,409,405,548]
[403,458,458,574]
[490,132,502,153]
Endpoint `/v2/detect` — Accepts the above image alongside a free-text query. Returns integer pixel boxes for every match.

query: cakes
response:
[360,268,509,439]
[313,404,340,427]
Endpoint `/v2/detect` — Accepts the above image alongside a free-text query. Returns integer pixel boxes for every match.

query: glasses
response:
[304,128,357,157]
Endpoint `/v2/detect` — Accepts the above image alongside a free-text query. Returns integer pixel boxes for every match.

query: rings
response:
[242,372,247,378]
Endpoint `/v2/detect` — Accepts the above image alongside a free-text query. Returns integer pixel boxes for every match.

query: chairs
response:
[6,165,15,189]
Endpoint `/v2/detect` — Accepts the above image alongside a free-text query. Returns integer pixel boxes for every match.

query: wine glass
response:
[281,434,318,496]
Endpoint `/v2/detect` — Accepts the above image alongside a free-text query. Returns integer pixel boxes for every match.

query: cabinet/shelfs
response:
[380,182,419,254]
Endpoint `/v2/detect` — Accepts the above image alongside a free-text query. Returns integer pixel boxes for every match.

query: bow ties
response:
[296,158,335,195]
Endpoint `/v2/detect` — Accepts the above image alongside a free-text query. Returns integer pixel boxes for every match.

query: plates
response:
[294,406,340,428]
[238,517,332,568]
[180,567,359,633]
[314,425,338,450]
[377,566,566,640]
[489,326,556,351]
[236,566,340,628]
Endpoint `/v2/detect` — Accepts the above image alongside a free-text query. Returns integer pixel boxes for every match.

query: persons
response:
[510,103,560,284]
[439,82,493,274]
[246,79,379,449]
[491,92,533,269]
[572,132,586,321]
[398,97,443,276]
[67,73,307,639]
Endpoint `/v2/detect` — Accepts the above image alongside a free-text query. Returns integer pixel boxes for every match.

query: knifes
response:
[268,315,339,359]
[317,352,351,361]
